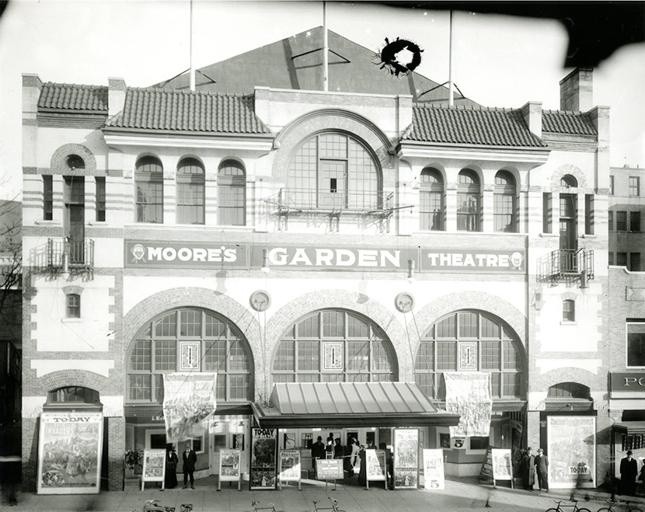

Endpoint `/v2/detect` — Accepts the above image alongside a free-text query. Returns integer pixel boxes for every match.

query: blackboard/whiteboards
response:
[491,448,513,480]
[219,449,241,480]
[142,449,166,482]
[280,450,301,481]
[366,449,387,481]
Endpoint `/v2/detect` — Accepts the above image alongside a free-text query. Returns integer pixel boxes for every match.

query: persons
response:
[311,432,393,487]
[165,450,179,488]
[255,441,274,466]
[519,447,536,491]
[533,449,549,492]
[182,443,197,489]
[620,450,638,496]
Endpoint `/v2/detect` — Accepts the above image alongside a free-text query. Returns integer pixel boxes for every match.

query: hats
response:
[527,447,532,450]
[317,436,322,440]
[538,449,544,452]
[626,451,633,455]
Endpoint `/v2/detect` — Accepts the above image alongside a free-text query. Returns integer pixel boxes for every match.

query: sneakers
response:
[181,486,196,489]
